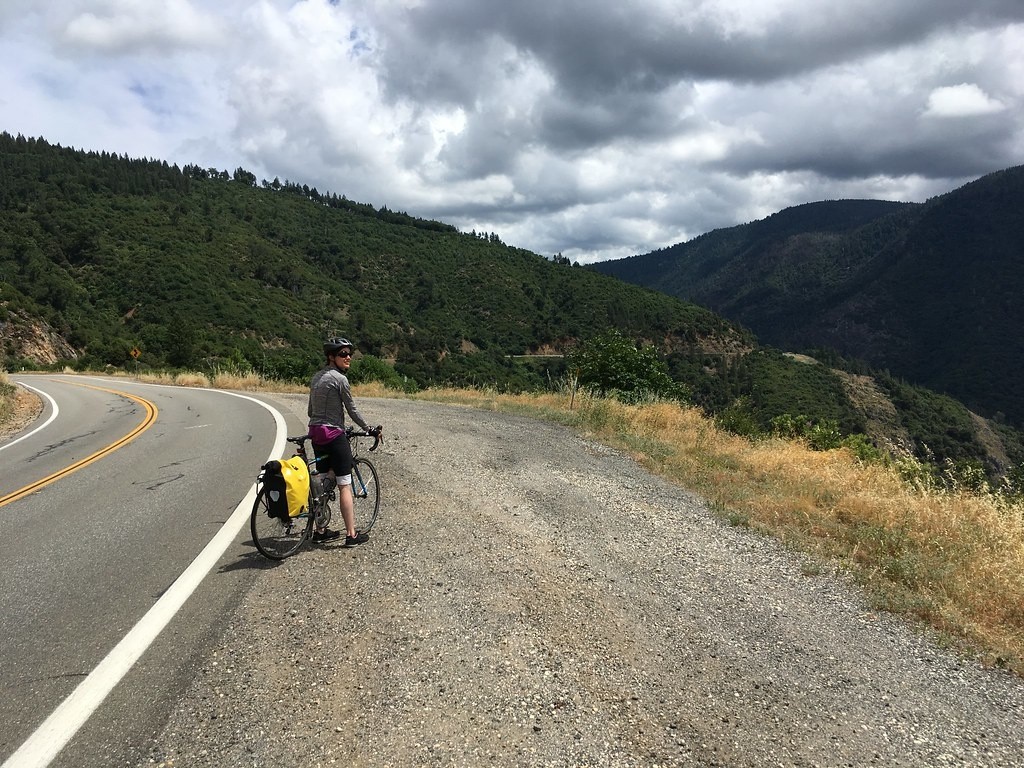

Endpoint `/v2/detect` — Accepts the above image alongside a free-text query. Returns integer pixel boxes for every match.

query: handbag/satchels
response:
[264,456,309,518]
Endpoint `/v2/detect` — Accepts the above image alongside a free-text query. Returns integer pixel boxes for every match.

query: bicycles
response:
[251,426,384,562]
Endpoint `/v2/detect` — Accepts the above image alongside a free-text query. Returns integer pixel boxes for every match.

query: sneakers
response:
[345,534,369,547]
[312,529,341,544]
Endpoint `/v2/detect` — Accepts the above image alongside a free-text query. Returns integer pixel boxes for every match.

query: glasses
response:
[333,350,354,358]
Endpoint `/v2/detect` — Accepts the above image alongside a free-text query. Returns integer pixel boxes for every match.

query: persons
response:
[307,337,380,545]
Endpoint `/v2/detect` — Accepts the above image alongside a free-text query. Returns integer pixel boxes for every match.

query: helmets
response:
[323,336,355,356]
